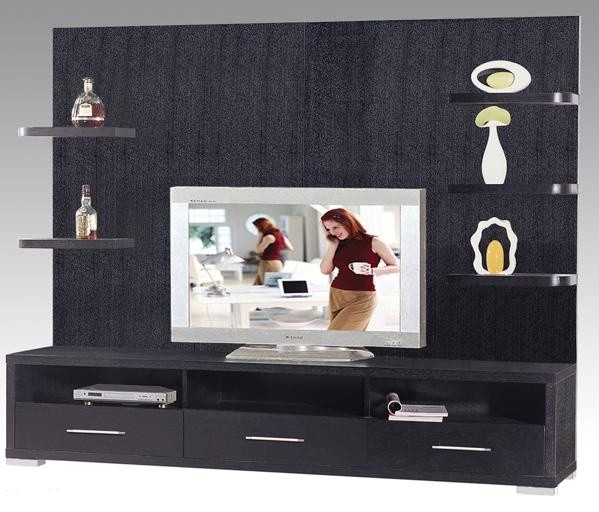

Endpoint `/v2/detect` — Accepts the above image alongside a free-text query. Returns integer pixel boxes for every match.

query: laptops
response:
[264,272,292,287]
[277,279,311,297]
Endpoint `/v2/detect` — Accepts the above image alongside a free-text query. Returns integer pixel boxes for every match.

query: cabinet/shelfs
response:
[443,90,580,289]
[15,125,138,252]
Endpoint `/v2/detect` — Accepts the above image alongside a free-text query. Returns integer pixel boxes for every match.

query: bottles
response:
[75,184,98,240]
[70,77,105,127]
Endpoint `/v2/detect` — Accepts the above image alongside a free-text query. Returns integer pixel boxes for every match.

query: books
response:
[384,392,448,417]
[387,413,443,423]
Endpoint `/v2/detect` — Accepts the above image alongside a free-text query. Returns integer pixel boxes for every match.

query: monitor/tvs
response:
[170,185,427,362]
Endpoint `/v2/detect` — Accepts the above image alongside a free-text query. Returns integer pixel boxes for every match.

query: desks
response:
[4,345,576,489]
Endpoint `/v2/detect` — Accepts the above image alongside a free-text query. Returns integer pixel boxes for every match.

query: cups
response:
[348,262,369,275]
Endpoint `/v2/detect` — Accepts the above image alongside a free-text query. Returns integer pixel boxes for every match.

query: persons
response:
[249,218,292,285]
[319,207,399,330]
